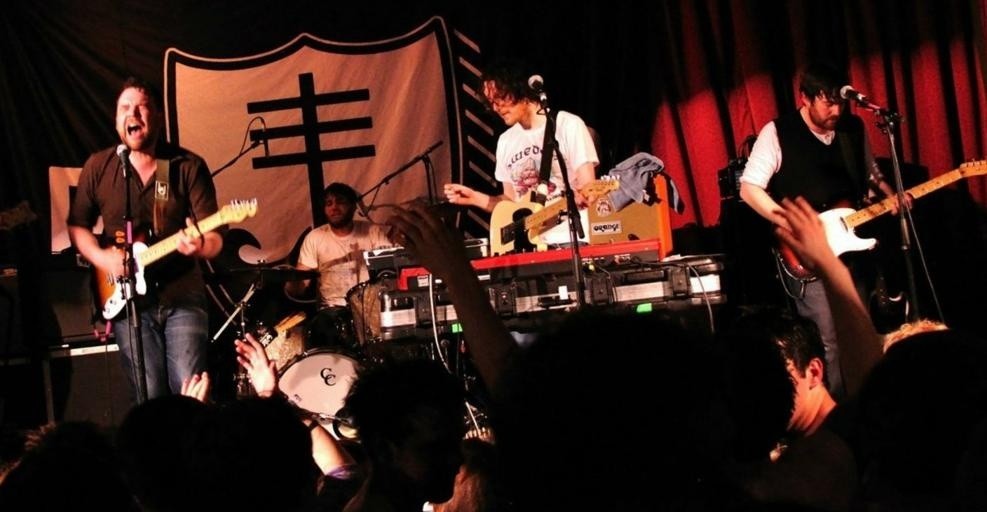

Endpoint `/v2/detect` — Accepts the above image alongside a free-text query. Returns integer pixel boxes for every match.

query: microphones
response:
[839,85,904,123]
[117,144,130,179]
[261,119,269,159]
[527,75,551,113]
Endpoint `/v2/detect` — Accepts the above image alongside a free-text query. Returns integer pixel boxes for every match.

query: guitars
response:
[489,175,620,259]
[89,197,258,321]
[775,157,986,282]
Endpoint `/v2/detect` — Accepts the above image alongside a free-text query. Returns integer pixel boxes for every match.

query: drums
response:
[278,347,361,441]
[344,281,386,365]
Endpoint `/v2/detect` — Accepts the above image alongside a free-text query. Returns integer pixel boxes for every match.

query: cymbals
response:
[209,266,321,282]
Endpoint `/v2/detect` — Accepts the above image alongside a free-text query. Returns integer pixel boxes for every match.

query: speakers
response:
[18,255,116,348]
[42,343,139,445]
